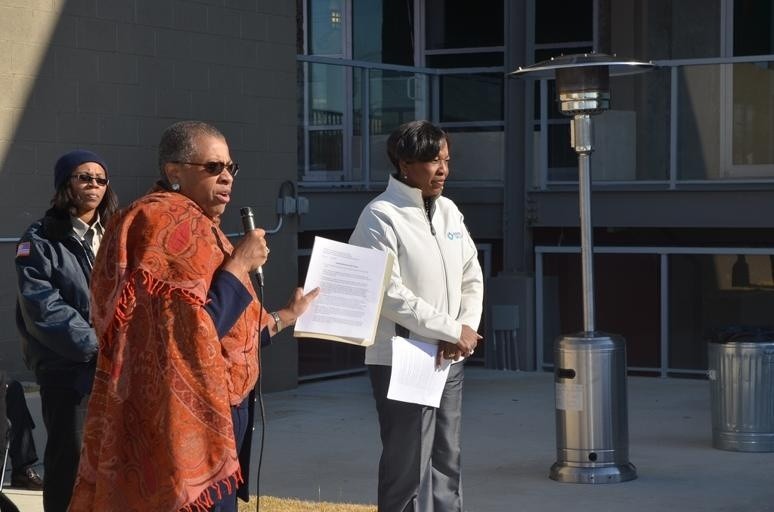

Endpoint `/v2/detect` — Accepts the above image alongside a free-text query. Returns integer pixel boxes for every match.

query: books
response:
[290,233,396,352]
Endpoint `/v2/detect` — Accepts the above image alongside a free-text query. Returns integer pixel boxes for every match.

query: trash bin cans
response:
[705,327,774,452]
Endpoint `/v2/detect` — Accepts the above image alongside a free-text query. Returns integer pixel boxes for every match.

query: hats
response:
[54,150,109,191]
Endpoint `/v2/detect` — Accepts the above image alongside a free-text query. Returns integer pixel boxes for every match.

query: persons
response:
[64,119,324,511]
[345,117,486,512]
[3,379,43,491]
[12,149,122,512]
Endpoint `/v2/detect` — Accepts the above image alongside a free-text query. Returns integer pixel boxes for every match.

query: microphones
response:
[239,206,265,288]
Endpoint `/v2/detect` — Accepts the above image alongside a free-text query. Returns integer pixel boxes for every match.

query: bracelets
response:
[270,311,283,334]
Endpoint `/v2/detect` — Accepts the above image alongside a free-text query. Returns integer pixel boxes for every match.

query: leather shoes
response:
[11,467,44,491]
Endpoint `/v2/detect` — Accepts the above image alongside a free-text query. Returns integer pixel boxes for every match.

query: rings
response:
[265,246,270,254]
[443,348,455,358]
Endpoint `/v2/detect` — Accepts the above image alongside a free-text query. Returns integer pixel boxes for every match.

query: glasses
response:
[69,172,110,186]
[165,159,240,178]
